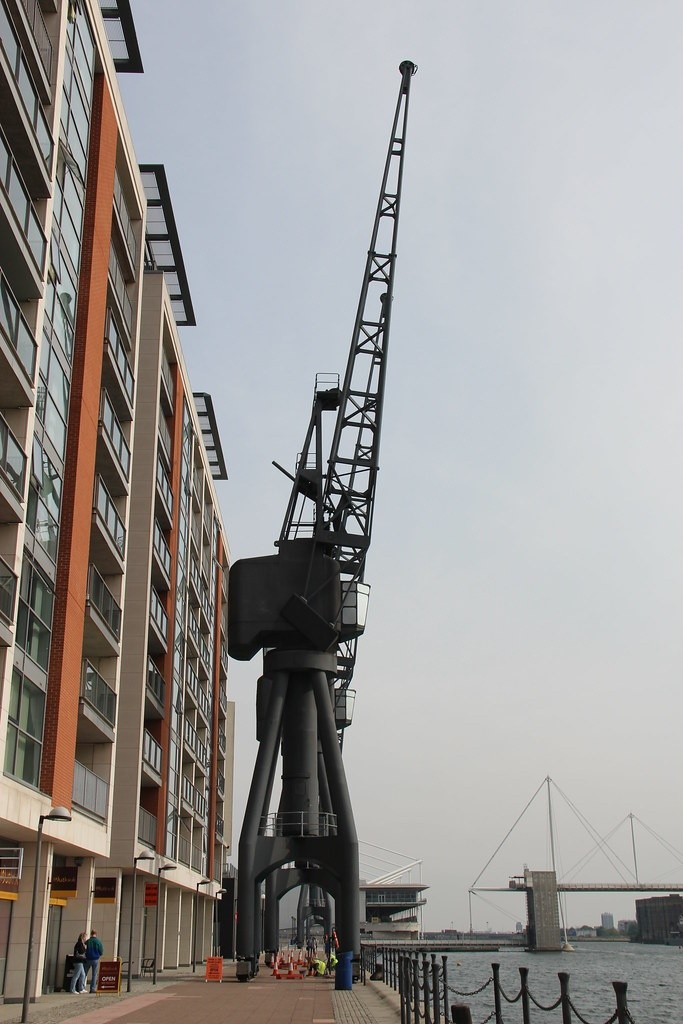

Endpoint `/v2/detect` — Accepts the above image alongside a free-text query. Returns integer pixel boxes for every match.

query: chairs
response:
[141,958,154,977]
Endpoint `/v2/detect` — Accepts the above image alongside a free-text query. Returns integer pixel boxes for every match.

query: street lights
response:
[215,889,227,956]
[127,850,154,993]
[153,863,177,985]
[261,893,266,955]
[291,916,296,940]
[21,806,72,1023]
[193,878,211,972]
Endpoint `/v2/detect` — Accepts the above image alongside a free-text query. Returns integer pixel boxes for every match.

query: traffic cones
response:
[305,966,315,977]
[287,957,294,976]
[270,959,278,976]
[275,950,294,969]
[297,949,303,965]
[302,954,308,967]
[269,953,275,969]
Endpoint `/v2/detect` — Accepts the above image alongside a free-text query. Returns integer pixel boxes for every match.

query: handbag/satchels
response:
[76,943,86,959]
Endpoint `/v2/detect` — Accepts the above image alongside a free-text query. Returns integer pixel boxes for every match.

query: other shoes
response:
[79,989,88,994]
[72,991,79,994]
[89,989,98,993]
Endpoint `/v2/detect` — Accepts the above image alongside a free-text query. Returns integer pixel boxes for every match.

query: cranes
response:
[225,57,420,991]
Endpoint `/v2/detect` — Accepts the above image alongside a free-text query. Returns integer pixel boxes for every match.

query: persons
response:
[293,934,303,949]
[326,953,338,971]
[70,932,89,994]
[306,957,329,977]
[307,936,318,957]
[84,929,104,992]
